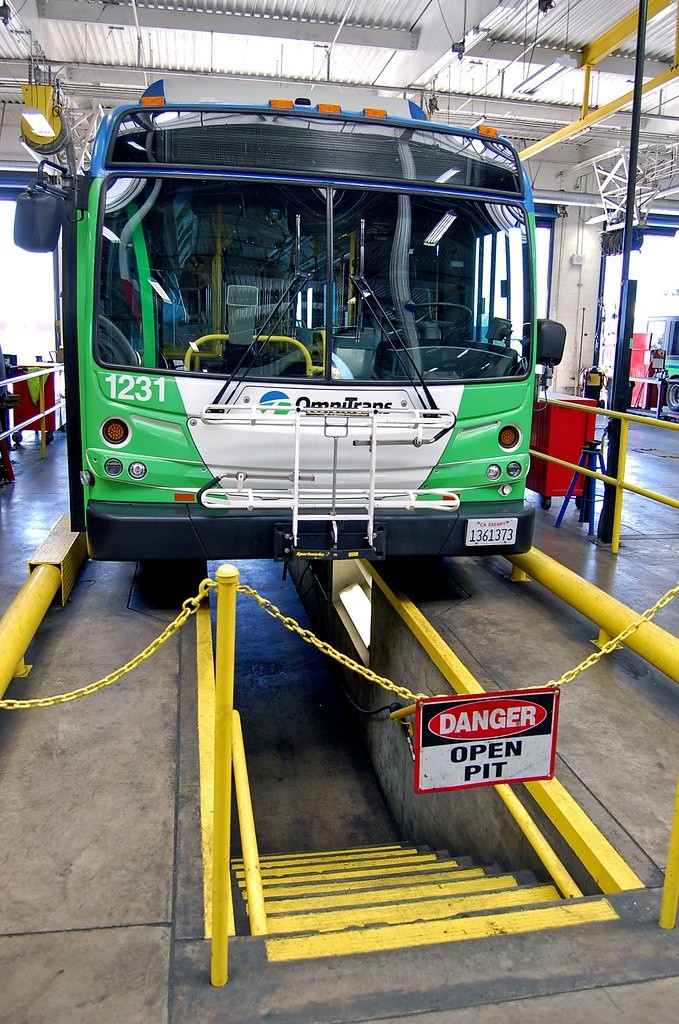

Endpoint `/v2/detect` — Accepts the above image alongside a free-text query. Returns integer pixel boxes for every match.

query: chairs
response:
[373,284,441,345]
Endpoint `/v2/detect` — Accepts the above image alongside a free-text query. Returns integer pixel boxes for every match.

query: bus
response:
[11,93,570,578]
